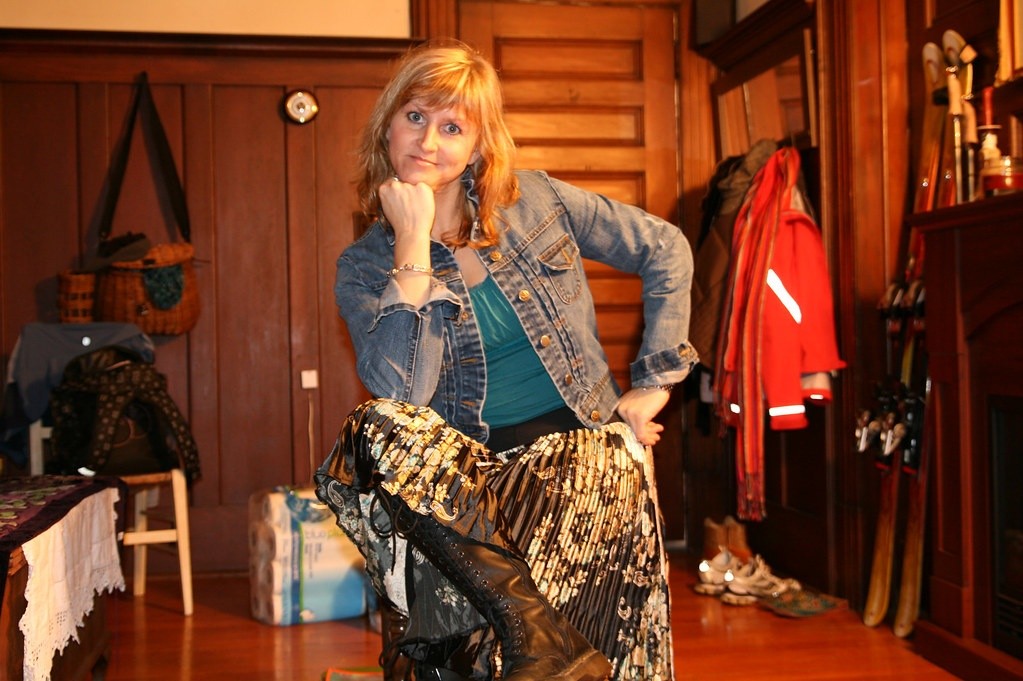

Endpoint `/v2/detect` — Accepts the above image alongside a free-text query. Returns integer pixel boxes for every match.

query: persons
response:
[334,42,698,680]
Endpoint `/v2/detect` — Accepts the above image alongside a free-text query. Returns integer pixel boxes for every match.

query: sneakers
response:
[718,557,801,606]
[694,544,743,596]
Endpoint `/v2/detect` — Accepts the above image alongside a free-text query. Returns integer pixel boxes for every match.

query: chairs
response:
[19,321,196,617]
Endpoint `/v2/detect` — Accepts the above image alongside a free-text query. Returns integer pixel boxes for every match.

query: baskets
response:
[56,267,97,323]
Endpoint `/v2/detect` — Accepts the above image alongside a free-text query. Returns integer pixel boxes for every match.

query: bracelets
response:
[640,380,674,392]
[384,259,437,278]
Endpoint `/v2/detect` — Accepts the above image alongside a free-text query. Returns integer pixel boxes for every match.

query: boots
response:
[370,484,613,680]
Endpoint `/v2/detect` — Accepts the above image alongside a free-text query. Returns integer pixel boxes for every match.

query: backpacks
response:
[82,357,203,491]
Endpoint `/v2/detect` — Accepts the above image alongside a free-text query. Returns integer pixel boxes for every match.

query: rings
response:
[391,176,399,181]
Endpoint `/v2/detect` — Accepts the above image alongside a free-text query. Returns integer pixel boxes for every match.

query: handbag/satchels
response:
[98,241,201,337]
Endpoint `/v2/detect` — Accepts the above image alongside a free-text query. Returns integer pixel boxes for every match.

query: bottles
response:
[983,156,1023,196]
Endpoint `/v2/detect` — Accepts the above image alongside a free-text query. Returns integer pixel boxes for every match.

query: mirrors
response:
[709,26,816,167]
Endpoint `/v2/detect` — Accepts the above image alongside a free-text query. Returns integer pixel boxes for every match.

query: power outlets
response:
[302,369,319,389]
